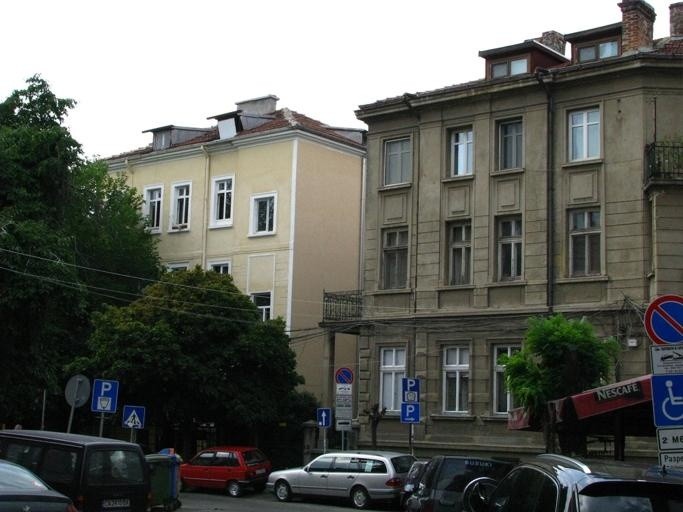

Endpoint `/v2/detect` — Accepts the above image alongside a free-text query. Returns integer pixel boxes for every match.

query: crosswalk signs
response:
[119,403,146,429]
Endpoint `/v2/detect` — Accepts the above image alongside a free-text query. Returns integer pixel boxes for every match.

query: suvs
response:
[264,448,417,508]
[482,449,682,512]
[401,455,521,512]
[0,424,150,511]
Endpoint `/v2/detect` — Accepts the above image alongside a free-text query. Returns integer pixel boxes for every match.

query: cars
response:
[174,445,272,495]
[396,455,426,504]
[0,459,80,512]
